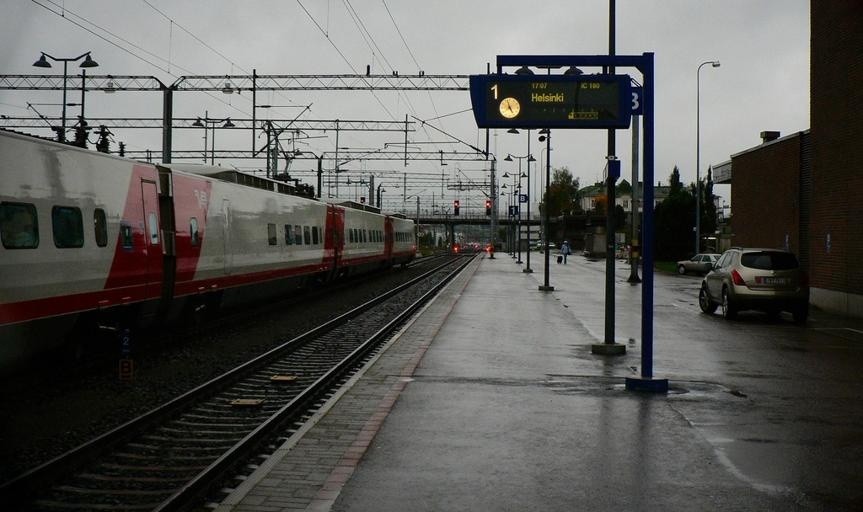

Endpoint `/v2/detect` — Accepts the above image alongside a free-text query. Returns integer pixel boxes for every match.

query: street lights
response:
[696,61,720,252]
[500,127,552,292]
[32,51,98,142]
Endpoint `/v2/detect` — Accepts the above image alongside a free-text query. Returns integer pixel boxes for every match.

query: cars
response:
[537,240,555,248]
[677,247,808,325]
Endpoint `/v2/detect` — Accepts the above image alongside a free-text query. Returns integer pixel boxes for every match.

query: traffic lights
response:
[485,201,491,215]
[454,246,459,252]
[454,200,459,215]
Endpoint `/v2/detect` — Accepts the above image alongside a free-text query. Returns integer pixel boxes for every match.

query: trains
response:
[1,130,417,375]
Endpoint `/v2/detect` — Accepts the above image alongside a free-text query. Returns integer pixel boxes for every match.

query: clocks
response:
[499,96,521,119]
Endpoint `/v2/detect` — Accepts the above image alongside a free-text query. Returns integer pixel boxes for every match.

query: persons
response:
[560,240,572,263]
